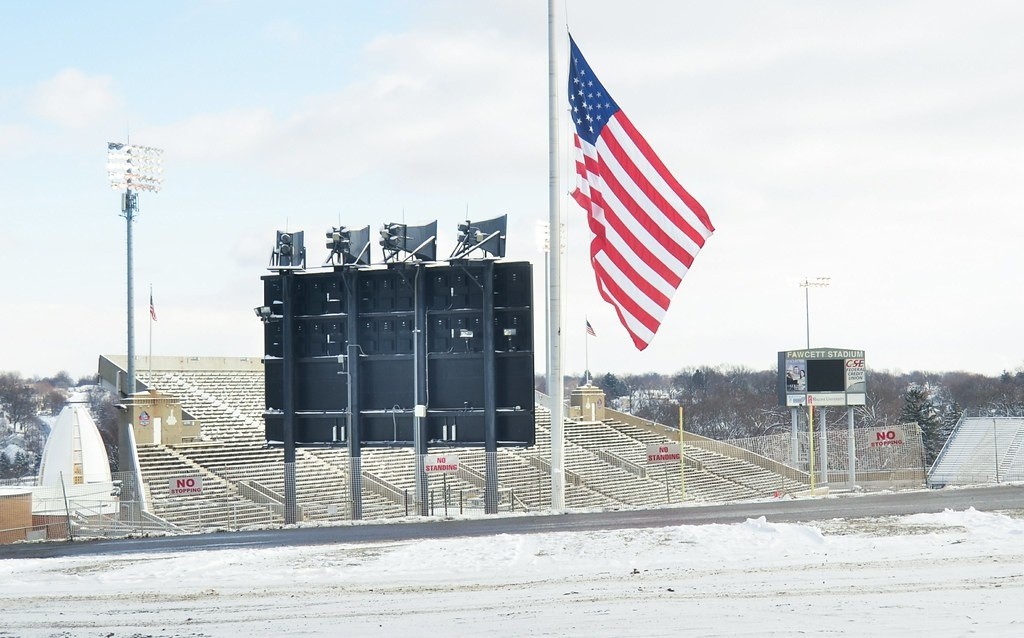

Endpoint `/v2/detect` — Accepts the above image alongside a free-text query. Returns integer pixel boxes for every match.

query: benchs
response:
[135,371,815,534]
[927,417,1024,483]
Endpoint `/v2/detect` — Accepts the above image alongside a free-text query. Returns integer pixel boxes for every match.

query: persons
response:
[788,366,805,391]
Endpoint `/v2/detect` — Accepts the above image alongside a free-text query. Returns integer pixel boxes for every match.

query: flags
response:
[150,293,156,321]
[563,33,716,353]
[585,320,596,337]
